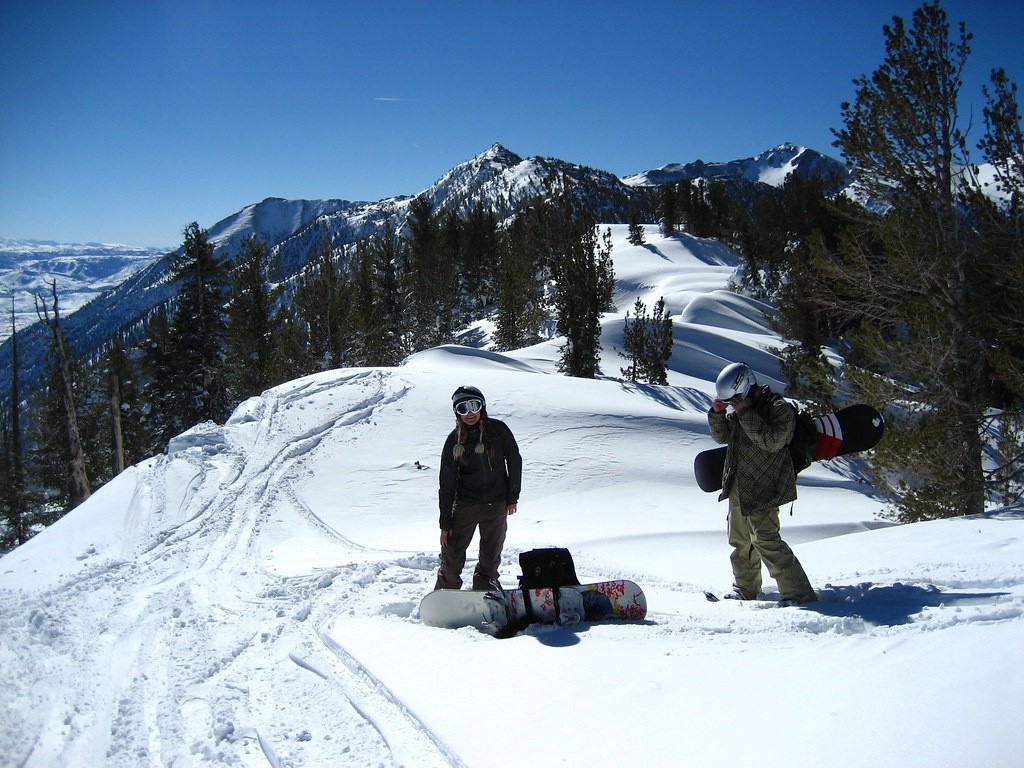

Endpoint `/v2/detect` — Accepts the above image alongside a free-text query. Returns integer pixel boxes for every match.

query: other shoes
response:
[724,584,759,600]
[779,591,819,607]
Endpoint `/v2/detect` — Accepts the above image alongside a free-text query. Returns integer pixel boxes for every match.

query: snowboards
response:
[693,403,886,494]
[418,577,648,629]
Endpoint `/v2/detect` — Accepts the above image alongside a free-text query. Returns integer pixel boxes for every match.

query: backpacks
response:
[517,547,582,627]
[762,393,817,475]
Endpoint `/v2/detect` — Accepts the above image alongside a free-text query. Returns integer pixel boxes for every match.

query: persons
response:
[435,385,522,593]
[707,362,819,605]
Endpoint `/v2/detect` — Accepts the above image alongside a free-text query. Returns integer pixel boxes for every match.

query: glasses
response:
[454,399,482,416]
[723,398,738,405]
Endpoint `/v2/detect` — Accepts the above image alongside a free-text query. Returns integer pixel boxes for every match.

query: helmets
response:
[716,362,757,400]
[451,386,486,412]
